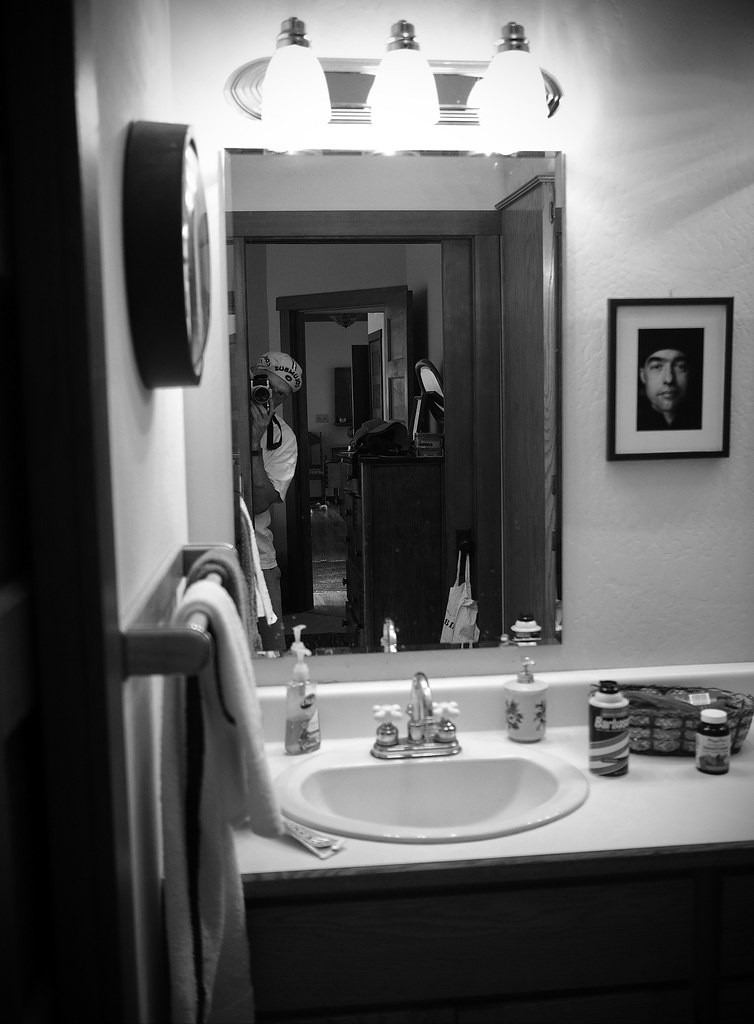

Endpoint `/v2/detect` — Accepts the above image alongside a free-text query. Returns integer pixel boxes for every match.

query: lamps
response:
[260,17,332,154]
[329,313,359,328]
[368,20,443,146]
[478,23,551,156]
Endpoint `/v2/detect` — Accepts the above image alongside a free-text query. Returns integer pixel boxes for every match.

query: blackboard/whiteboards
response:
[332,366,353,426]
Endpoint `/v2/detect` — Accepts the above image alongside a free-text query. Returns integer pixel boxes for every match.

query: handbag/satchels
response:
[439,551,480,644]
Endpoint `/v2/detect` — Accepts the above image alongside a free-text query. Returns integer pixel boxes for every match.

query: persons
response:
[250,352,302,653]
[638,332,701,430]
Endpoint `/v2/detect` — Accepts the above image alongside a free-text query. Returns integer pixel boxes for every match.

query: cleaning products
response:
[504,659,546,742]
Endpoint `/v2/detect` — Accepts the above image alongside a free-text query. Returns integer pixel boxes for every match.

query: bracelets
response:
[252,448,263,456]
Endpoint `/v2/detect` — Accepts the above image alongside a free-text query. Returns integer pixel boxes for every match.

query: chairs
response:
[308,431,327,504]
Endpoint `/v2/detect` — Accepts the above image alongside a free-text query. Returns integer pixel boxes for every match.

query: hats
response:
[255,352,302,397]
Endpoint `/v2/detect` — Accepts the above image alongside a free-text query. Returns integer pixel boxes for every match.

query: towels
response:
[241,496,280,626]
[164,548,285,1023]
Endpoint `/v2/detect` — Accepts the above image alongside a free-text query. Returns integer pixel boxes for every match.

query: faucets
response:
[382,616,399,652]
[370,671,463,761]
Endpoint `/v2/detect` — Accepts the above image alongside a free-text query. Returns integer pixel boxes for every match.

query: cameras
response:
[250,374,273,411]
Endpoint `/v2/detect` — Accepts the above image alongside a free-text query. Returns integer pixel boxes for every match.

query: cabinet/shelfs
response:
[338,451,445,646]
[244,863,754,1024]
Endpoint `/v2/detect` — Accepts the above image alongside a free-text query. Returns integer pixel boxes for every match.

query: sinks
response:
[271,741,588,843]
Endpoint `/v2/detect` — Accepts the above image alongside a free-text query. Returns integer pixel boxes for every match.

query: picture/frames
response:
[606,297,736,460]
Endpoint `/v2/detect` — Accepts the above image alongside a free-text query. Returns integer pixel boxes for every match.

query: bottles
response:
[587,679,630,778]
[697,707,733,775]
[510,614,543,647]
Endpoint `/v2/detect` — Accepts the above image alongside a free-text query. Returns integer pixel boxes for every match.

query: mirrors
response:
[219,144,566,658]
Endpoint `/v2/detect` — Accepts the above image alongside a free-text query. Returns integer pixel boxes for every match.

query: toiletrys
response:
[289,624,312,658]
[589,680,632,777]
[273,811,346,861]
[286,649,320,754]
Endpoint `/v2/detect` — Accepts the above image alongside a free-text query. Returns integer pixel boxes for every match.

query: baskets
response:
[622,684,754,755]
